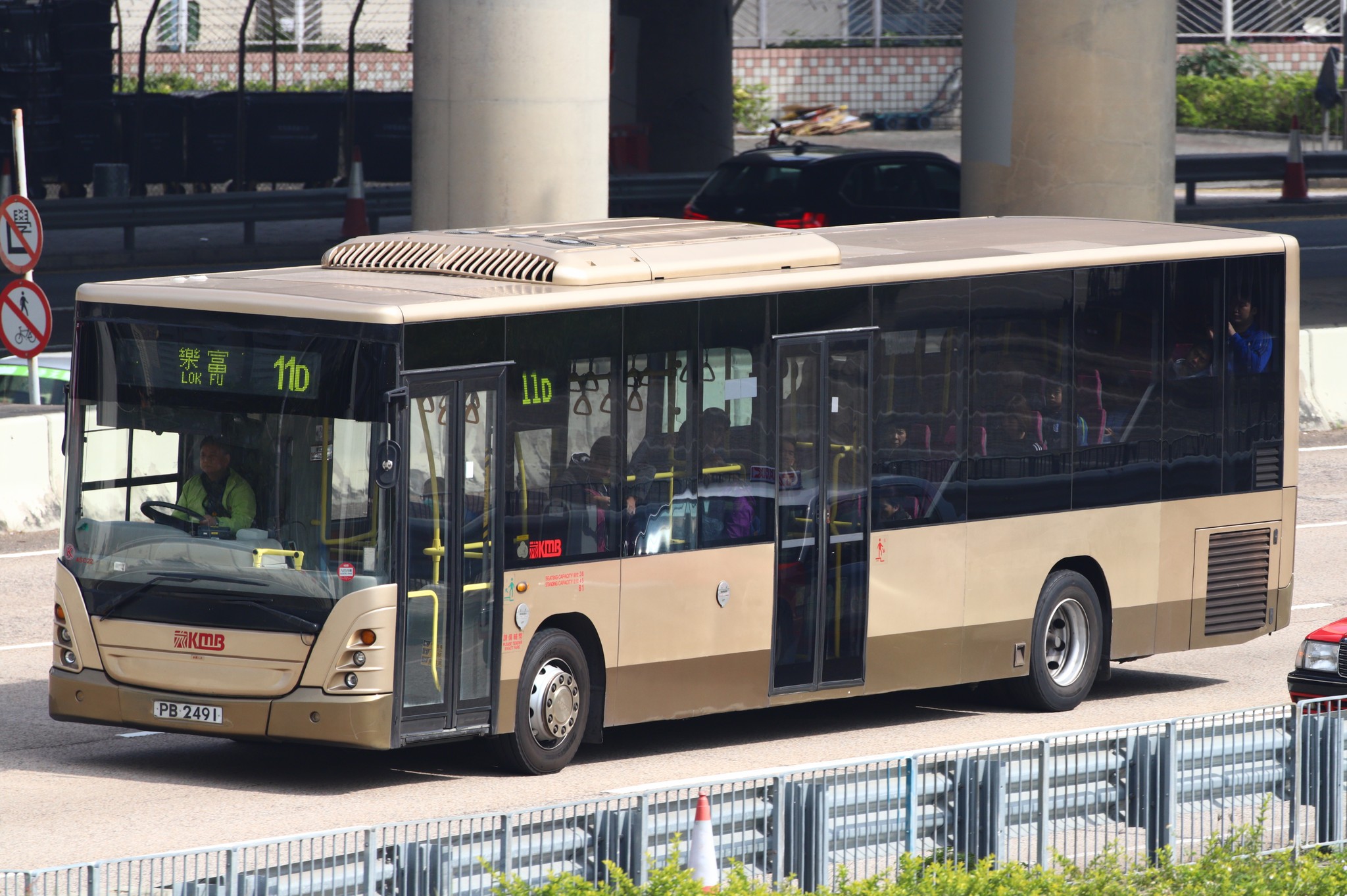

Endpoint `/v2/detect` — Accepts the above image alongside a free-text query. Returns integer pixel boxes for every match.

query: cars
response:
[1286,617,1347,714]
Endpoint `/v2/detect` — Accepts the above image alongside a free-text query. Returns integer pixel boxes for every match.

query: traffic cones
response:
[339,143,376,244]
[689,793,724,894]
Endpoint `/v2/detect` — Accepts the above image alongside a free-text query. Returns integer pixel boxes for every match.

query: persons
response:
[551,435,656,552]
[879,489,912,521]
[782,433,801,531]
[1039,379,1088,450]
[675,407,732,462]
[702,453,759,538]
[872,415,928,496]
[1103,378,1134,444]
[1164,337,1214,386]
[785,354,855,484]
[986,392,1043,455]
[170,436,256,539]
[1206,286,1272,376]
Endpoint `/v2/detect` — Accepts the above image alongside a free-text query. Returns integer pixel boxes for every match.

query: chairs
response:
[838,368,1107,523]
[229,447,292,537]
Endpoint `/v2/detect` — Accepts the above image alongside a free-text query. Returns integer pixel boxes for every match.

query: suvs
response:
[674,138,960,233]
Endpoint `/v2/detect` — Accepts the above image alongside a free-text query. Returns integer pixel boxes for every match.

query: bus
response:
[40,217,1302,776]
